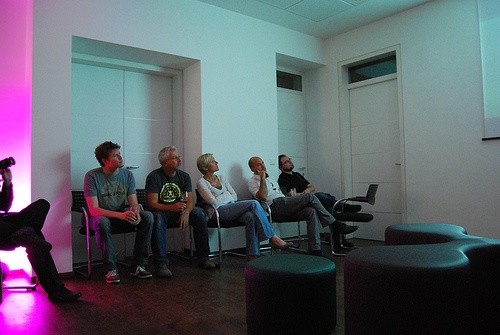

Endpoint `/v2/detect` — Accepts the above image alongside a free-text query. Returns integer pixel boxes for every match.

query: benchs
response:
[343,223,500,335]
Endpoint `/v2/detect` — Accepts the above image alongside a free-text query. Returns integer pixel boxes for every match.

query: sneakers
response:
[129,264,153,278]
[105,270,121,283]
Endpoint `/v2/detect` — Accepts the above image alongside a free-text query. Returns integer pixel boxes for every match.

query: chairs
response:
[0,211,40,289]
[71,183,379,280]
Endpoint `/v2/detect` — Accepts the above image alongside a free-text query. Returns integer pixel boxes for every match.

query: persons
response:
[278,154,362,246]
[249,156,360,263]
[0,168,82,306]
[196,153,293,262]
[145,145,215,276]
[84,142,154,283]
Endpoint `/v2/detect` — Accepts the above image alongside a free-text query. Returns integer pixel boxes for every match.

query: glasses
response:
[164,155,182,161]
[281,157,291,166]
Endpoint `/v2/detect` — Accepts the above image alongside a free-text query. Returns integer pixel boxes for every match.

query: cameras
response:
[0,156,16,171]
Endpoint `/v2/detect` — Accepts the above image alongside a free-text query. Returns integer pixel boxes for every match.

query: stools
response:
[243,253,337,335]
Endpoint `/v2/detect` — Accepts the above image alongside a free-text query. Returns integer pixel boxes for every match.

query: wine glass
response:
[178,191,188,215]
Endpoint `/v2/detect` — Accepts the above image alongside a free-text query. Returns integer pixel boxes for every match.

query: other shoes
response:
[268,235,289,250]
[151,262,172,278]
[49,285,82,303]
[334,243,353,255]
[307,250,323,257]
[247,255,257,262]
[13,226,52,251]
[342,203,362,213]
[196,255,216,270]
[334,222,360,236]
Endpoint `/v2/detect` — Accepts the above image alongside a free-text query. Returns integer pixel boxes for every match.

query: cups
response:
[291,188,297,196]
[130,207,138,216]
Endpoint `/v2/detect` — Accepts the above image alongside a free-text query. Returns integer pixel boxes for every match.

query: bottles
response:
[0,156,16,170]
